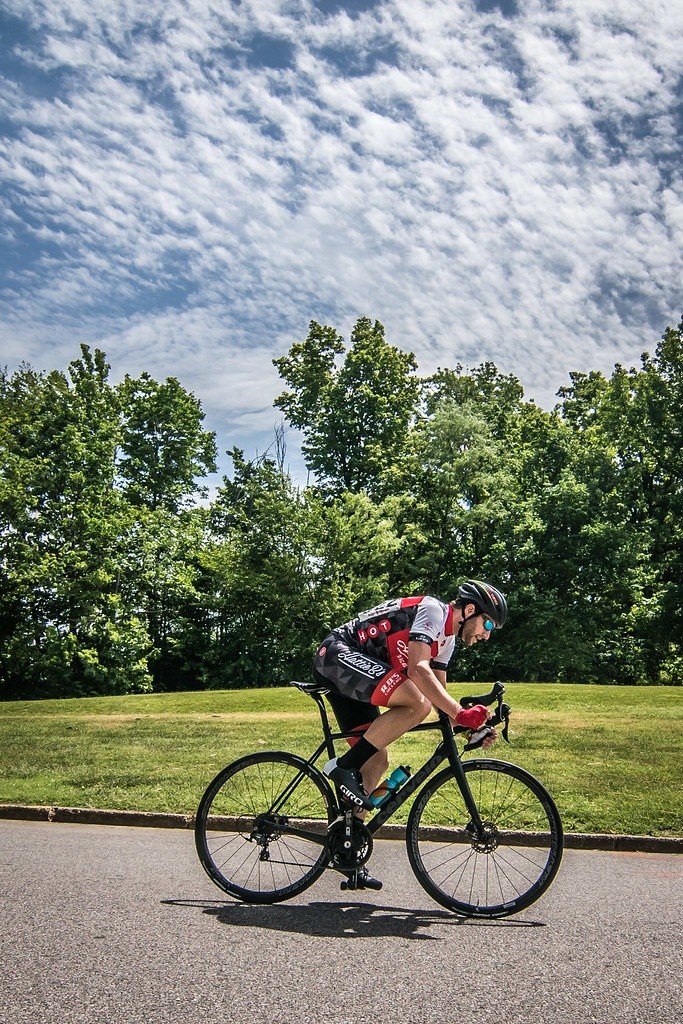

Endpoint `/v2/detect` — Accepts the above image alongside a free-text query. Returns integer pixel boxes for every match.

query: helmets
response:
[459,580,508,629]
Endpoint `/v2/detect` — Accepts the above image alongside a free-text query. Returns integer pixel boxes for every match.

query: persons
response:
[311,578,508,892]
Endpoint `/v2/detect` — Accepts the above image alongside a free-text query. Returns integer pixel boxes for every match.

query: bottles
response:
[368,765,412,810]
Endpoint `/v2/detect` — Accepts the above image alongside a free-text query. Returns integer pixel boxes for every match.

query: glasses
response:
[481,613,495,630]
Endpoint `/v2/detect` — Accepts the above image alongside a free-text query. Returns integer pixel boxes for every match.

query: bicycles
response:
[193,680,564,920]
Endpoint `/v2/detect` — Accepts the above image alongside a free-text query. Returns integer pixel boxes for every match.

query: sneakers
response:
[334,855,384,891]
[323,756,374,811]
